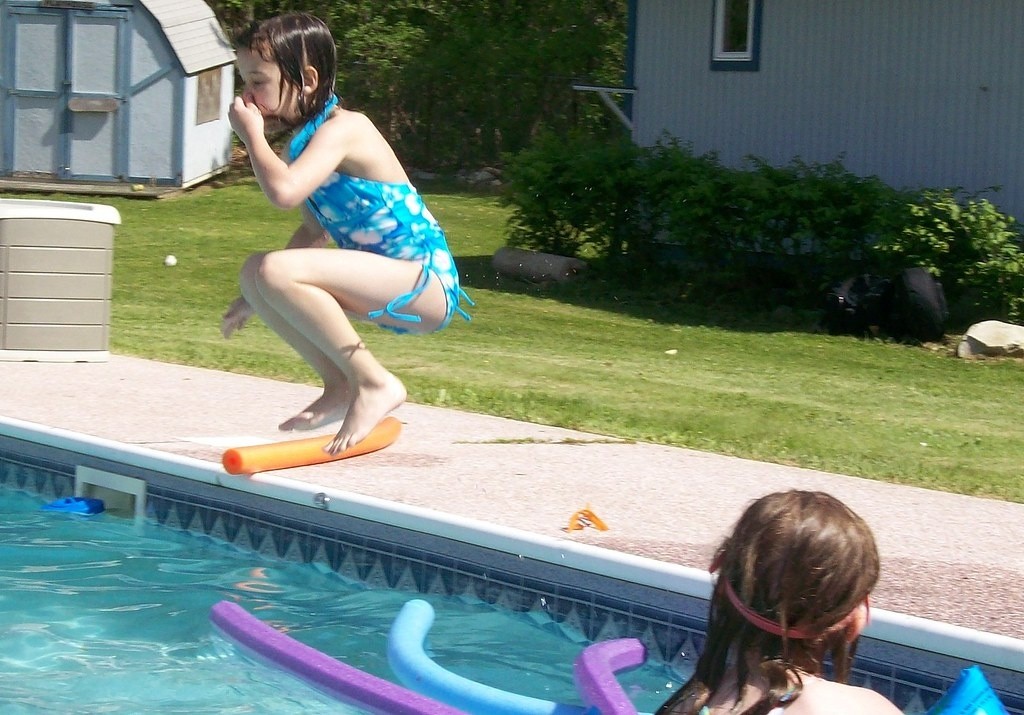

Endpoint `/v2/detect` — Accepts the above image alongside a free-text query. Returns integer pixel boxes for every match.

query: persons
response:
[218,12,473,456]
[656,491,902,715]
